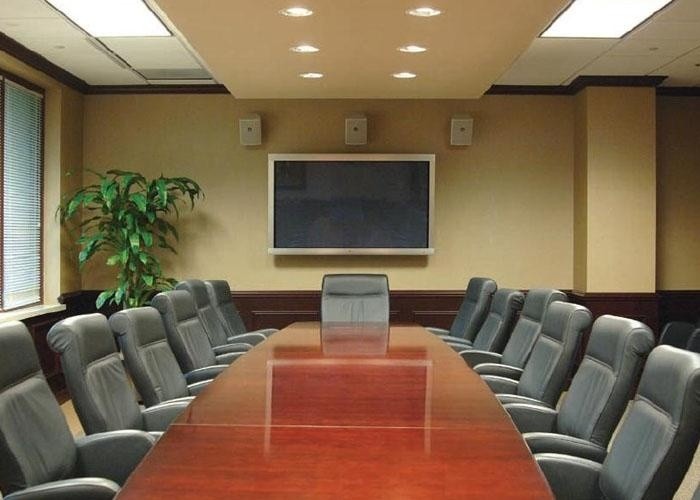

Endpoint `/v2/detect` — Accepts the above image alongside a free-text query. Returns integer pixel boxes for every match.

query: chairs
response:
[423,276,594,409]
[1,319,154,500]
[500,315,656,462]
[319,273,391,323]
[47,280,282,438]
[534,345,700,499]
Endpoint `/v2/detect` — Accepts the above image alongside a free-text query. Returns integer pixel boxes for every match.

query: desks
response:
[110,315,556,499]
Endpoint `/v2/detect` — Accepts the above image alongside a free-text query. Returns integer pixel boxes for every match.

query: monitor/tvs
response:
[267,152,435,256]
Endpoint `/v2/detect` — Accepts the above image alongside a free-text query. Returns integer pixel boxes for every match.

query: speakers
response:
[450,118,473,146]
[345,119,367,146]
[239,117,261,145]
